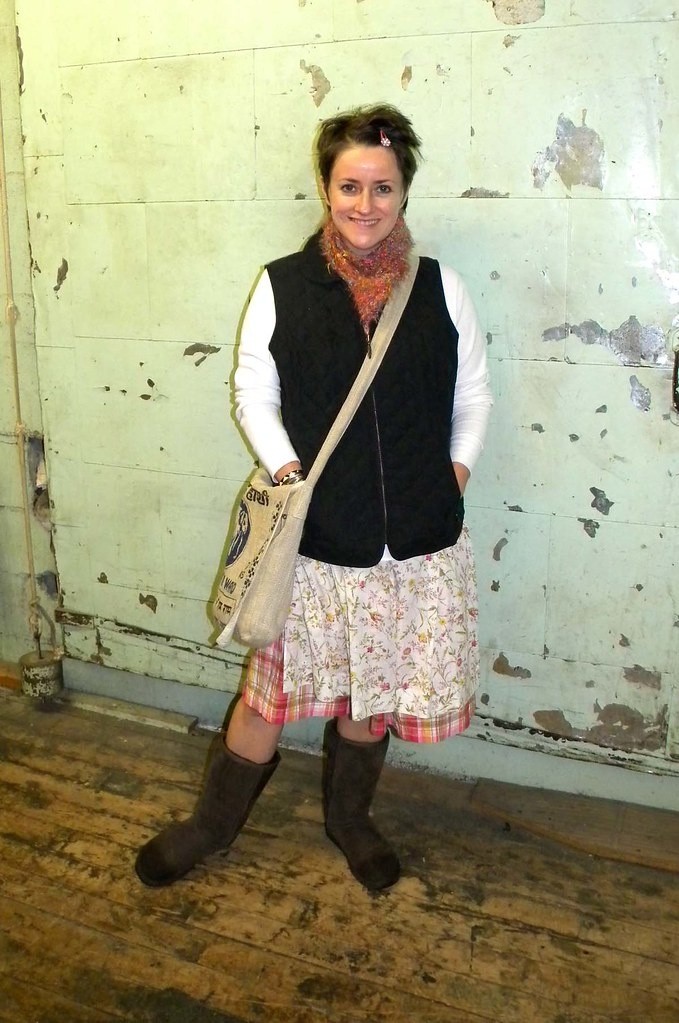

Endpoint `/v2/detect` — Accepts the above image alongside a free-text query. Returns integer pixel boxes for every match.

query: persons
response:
[132,105,495,891]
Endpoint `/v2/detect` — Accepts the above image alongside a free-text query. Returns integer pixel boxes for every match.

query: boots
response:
[136,731,282,888]
[324,731,403,893]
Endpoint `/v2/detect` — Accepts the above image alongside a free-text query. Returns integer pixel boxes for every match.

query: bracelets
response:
[279,470,304,486]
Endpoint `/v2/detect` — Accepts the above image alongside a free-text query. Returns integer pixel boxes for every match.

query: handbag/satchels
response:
[210,468,314,649]
